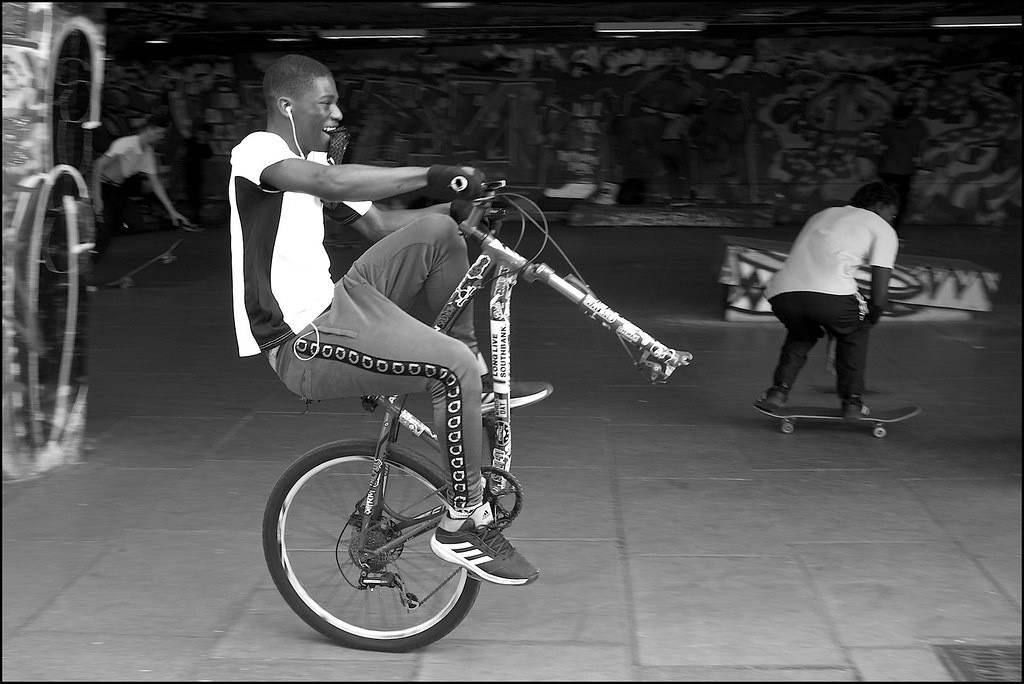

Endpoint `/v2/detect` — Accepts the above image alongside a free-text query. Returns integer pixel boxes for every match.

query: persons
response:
[229,63,553,585]
[74,116,190,291]
[660,112,698,200]
[765,181,900,420]
[175,122,214,232]
[880,91,928,241]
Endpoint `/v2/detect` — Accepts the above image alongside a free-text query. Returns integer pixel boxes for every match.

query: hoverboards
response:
[261,175,693,653]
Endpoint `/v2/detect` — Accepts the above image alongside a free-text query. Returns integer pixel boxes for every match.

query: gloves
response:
[427,162,487,199]
[450,200,489,234]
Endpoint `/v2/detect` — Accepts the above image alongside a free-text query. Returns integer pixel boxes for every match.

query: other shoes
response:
[181,224,204,232]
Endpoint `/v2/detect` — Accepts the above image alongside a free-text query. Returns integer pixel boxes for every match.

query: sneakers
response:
[843,401,876,418]
[430,502,540,586]
[481,372,554,415]
[758,387,787,412]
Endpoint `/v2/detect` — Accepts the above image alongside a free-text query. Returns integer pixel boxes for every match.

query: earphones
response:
[285,106,293,119]
